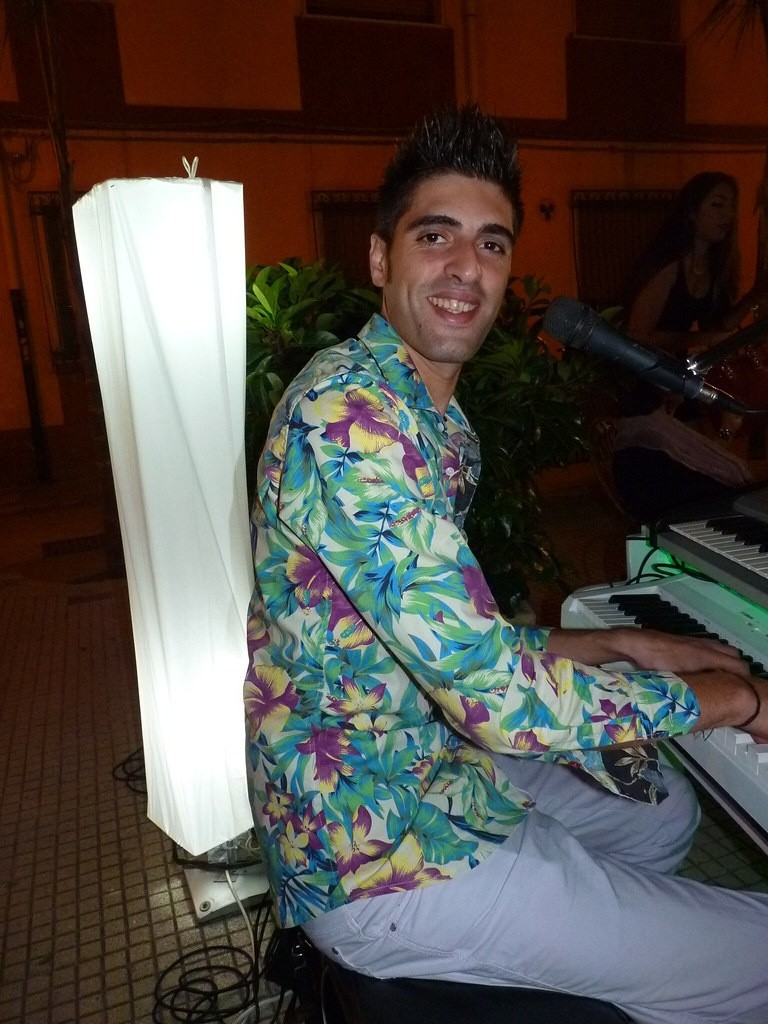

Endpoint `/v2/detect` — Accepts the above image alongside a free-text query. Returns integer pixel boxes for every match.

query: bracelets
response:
[731,673,760,728]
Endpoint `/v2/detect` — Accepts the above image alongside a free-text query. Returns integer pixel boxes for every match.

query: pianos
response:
[560,487,768,883]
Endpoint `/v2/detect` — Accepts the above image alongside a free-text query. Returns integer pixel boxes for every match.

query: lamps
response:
[69,157,269,924]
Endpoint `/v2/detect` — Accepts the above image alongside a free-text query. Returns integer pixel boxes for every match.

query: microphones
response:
[542,296,748,416]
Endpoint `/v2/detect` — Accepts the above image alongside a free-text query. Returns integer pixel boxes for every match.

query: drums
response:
[703,318,767,438]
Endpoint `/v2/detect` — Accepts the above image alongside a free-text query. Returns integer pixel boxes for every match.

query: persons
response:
[245,104,768,1024]
[611,170,743,416]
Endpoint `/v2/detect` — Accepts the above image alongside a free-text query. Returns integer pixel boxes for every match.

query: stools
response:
[264,928,634,1024]
